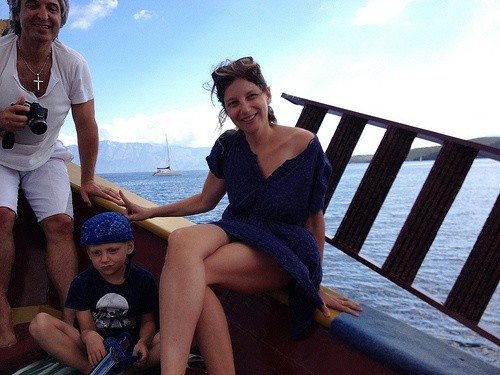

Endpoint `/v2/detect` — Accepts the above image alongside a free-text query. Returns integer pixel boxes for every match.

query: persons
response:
[118,56,363,375]
[29,211,160,374]
[0,0,123,348]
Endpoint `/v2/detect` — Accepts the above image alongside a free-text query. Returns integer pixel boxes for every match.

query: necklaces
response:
[16,39,52,90]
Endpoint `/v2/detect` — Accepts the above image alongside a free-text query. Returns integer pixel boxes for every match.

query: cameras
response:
[15,100,48,135]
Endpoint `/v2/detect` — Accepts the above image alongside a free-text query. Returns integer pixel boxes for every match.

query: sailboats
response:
[152,131,184,177]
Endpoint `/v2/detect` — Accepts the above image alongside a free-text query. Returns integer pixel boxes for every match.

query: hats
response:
[78,211,134,246]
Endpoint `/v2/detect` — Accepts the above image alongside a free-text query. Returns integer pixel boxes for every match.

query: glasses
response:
[212,56,253,82]
